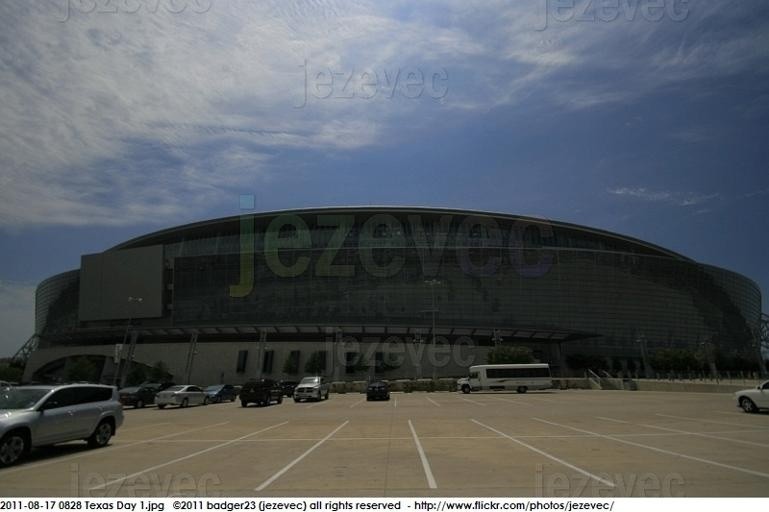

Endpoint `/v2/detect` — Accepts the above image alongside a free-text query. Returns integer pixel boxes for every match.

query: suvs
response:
[240,379,283,405]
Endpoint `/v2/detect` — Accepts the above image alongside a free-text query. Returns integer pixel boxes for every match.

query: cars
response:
[154,384,210,407]
[294,377,329,402]
[118,387,157,408]
[734,381,769,412]
[203,385,237,402]
[368,380,391,399]
[284,381,299,399]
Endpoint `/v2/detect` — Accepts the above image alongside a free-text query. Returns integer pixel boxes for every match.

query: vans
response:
[0,384,124,467]
[456,364,553,394]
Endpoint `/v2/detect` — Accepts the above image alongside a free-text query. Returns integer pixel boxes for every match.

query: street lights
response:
[115,297,145,382]
